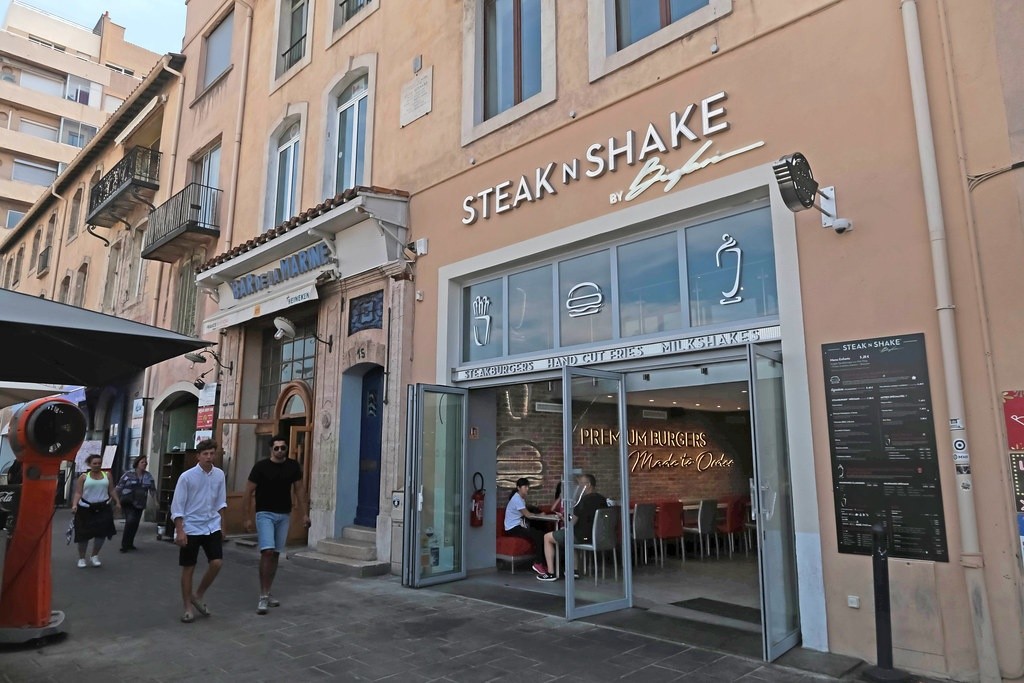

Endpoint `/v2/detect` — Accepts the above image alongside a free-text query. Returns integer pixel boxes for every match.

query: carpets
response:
[668,597,761,625]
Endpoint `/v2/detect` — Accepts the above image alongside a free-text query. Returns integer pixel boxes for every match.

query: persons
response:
[114,454,160,555]
[244,435,312,613]
[3,459,22,539]
[171,438,228,622]
[71,454,122,568]
[503,473,608,581]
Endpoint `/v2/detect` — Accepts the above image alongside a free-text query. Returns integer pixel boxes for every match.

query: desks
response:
[530,501,727,578]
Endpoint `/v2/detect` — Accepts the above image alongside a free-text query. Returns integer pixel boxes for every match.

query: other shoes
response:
[536,572,557,582]
[77,558,87,568]
[90,555,102,567]
[191,591,211,616]
[120,545,137,552]
[181,612,195,623]
[532,562,548,575]
[258,594,269,612]
[564,570,580,579]
[267,591,280,607]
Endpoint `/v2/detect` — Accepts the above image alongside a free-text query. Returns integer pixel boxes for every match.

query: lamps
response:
[773,152,838,227]
[184,350,233,376]
[194,368,213,390]
[274,317,333,353]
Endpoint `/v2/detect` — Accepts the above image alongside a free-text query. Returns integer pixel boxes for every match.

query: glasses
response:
[274,445,287,451]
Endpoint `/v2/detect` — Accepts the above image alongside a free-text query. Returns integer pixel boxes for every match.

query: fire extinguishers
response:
[471,488,486,527]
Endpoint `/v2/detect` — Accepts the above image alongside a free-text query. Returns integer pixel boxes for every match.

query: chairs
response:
[496,506,553,575]
[573,499,757,589]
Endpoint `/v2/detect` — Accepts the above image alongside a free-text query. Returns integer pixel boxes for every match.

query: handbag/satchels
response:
[90,502,116,538]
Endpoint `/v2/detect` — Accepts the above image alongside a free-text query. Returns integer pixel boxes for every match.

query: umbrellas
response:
[1,288,219,385]
[65,513,75,545]
